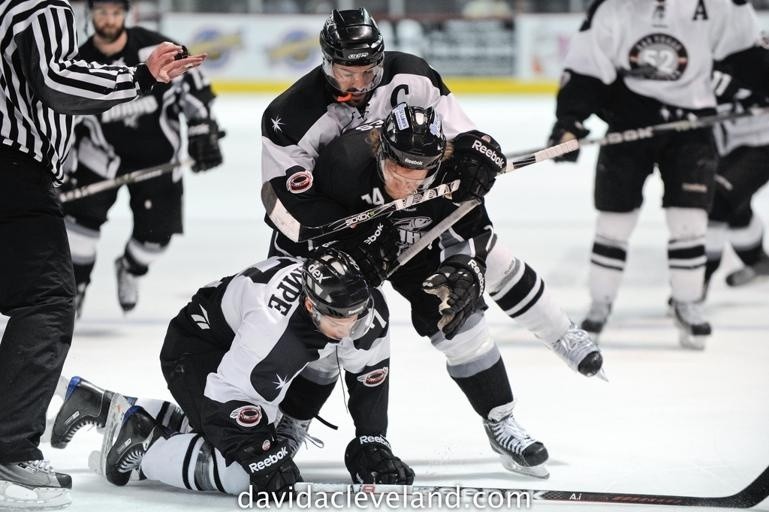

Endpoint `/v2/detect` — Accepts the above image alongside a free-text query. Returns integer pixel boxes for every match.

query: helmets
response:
[319,6,384,93]
[303,240,377,340]
[380,101,445,200]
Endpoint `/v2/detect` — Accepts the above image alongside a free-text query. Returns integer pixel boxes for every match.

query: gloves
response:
[237,424,304,504]
[423,253,486,338]
[446,130,504,203]
[187,134,222,175]
[547,122,590,162]
[345,437,413,488]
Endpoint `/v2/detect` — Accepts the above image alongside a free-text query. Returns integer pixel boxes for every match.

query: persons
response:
[260,5,608,383]
[665,1,769,304]
[269,98,554,469]
[544,0,758,344]
[1,0,208,492]
[63,0,228,317]
[47,244,417,505]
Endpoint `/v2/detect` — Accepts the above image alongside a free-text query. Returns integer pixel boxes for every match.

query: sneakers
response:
[726,261,765,283]
[485,416,549,467]
[118,266,137,309]
[276,413,310,457]
[669,295,717,337]
[547,321,602,374]
[0,378,158,487]
[581,298,610,328]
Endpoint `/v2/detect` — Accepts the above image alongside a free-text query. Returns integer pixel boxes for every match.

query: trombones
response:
[261,138,579,244]
